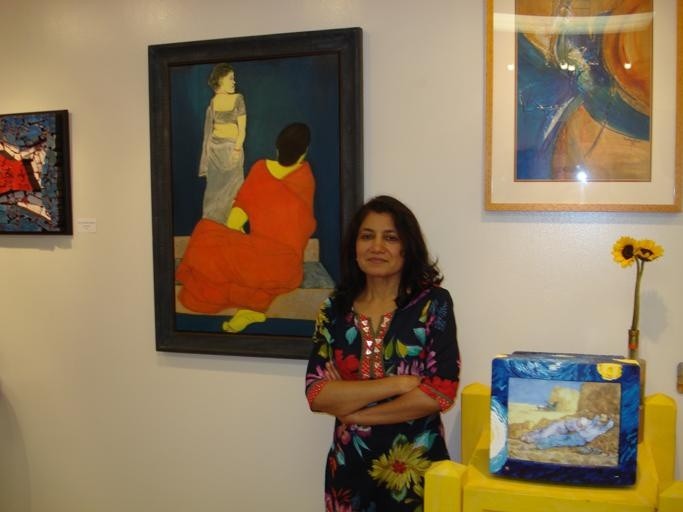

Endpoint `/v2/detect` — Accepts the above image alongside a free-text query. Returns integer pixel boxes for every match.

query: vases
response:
[620,330,645,443]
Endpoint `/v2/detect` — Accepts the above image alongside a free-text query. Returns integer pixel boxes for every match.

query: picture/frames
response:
[144,25,365,362]
[0,108,73,236]
[483,0,682,214]
[488,354,640,488]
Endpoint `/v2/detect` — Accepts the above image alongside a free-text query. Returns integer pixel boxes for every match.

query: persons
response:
[164,121,318,334]
[196,65,246,226]
[303,194,461,512]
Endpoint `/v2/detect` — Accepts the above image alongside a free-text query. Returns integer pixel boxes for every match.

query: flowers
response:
[611,232,666,331]
